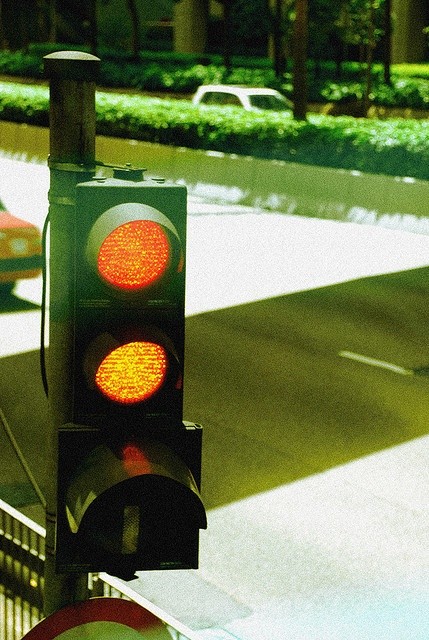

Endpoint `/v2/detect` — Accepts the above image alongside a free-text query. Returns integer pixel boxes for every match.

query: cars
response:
[193,84,294,111]
[0,199,42,291]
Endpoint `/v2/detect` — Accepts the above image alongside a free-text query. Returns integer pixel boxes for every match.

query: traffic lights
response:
[54,166,207,582]
